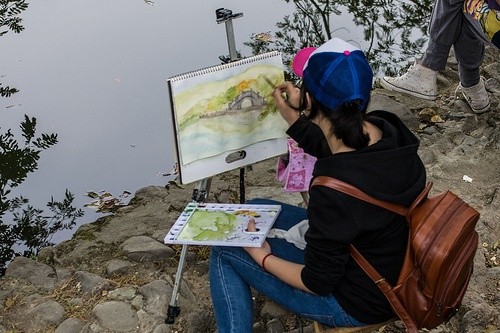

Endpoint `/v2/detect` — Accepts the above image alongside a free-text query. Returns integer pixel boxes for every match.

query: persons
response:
[380,0,500,113]
[209,38,426,333]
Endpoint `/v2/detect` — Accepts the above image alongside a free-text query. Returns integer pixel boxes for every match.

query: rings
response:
[296,90,300,93]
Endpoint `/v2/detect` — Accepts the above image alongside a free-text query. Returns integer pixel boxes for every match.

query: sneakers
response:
[380,64,438,100]
[458,75,490,114]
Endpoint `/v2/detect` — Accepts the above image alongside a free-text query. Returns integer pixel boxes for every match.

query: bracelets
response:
[262,252,273,271]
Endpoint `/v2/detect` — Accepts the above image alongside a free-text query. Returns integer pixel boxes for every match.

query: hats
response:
[291,37,373,110]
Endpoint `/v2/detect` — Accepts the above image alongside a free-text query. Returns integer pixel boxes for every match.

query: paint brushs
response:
[262,74,286,100]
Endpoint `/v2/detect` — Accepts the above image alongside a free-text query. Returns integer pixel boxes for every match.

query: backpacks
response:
[309,174,481,332]
